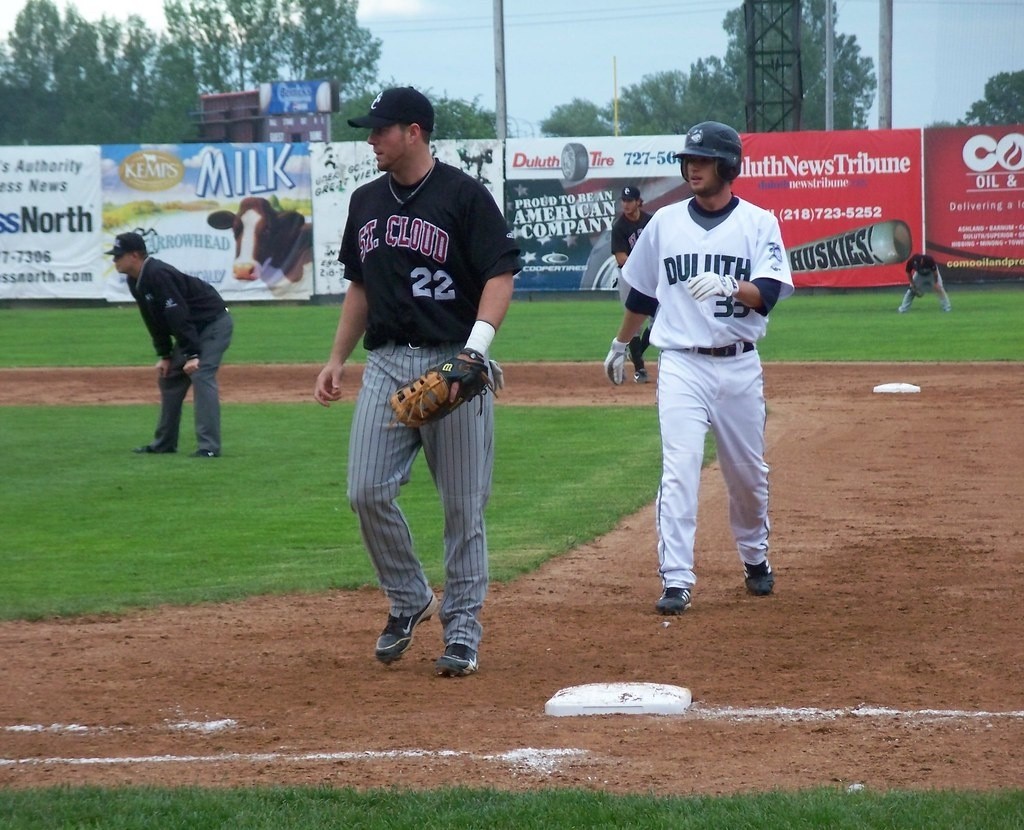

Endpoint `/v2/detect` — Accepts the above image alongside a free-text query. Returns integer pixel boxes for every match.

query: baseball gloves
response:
[386,357,488,428]
[910,286,924,298]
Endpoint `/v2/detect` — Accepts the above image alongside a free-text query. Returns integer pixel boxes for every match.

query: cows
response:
[207,198,313,287]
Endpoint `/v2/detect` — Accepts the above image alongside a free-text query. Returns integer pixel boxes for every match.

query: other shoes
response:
[633,369,648,385]
[192,448,218,458]
[132,444,154,454]
[627,341,650,362]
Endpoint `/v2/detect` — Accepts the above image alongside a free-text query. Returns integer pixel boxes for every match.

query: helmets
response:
[674,121,743,185]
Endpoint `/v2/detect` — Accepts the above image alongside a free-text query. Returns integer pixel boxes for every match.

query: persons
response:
[611,186,661,384]
[605,122,794,613]
[898,254,951,312]
[315,86,521,677]
[104,232,233,456]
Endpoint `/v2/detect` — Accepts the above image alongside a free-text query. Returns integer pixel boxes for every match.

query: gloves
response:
[604,337,631,385]
[488,361,505,392]
[688,272,740,302]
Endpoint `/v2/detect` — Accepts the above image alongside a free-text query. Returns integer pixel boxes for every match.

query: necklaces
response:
[389,157,436,203]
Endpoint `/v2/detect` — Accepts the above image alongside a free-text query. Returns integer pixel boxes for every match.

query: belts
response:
[691,342,756,357]
[391,341,426,352]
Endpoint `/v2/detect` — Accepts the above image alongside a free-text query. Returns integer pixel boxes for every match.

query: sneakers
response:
[376,591,437,663]
[436,642,478,677]
[743,558,774,595]
[655,585,691,615]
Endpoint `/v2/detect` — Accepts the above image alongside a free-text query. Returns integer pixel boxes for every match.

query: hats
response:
[912,254,921,270]
[348,86,434,133]
[618,186,640,201]
[105,232,145,256]
